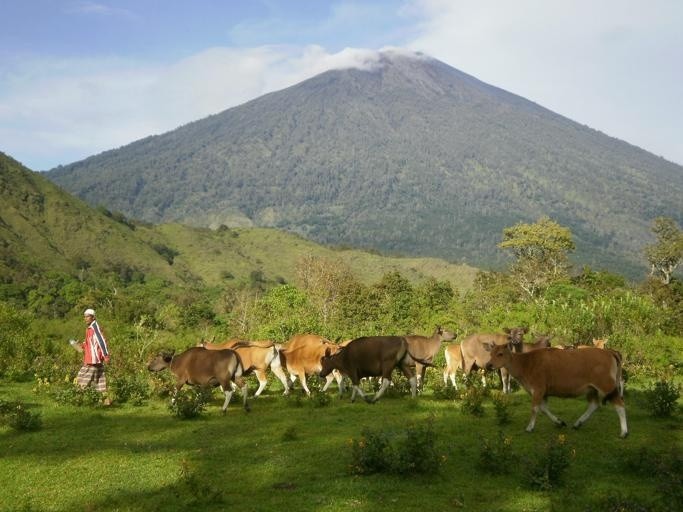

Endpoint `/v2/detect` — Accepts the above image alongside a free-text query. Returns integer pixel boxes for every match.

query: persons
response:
[74,308,112,406]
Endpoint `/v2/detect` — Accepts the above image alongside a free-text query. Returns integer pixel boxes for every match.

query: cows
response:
[459,325,528,394]
[147,347,261,414]
[319,334,439,400]
[276,333,343,395]
[482,340,629,439]
[522,336,608,353]
[443,344,486,389]
[406,324,457,389]
[195,336,288,397]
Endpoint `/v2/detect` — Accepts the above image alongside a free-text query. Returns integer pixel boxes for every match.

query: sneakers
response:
[104,398,111,405]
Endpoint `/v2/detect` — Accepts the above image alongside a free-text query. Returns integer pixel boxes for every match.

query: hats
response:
[85,308,96,316]
